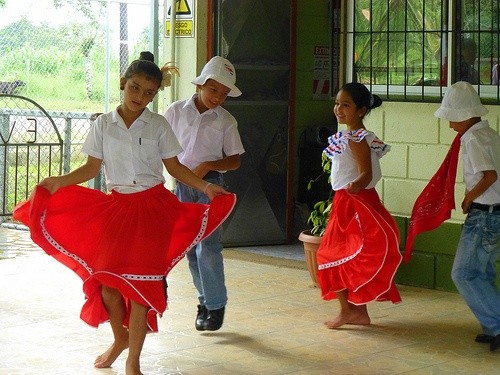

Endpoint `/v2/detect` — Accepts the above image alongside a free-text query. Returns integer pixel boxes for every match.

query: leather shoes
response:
[475,334,496,343]
[203,306,224,330]
[195,304,207,330]
[490,334,500,351]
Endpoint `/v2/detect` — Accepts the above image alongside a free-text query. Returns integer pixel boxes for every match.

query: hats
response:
[434,81,490,123]
[190,56,242,97]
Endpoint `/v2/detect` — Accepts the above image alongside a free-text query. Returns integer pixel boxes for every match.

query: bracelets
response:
[203,183,212,194]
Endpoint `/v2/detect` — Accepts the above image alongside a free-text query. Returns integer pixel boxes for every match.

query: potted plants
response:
[298,152,332,288]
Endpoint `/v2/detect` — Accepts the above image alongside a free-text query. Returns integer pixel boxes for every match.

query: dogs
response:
[0,79,26,95]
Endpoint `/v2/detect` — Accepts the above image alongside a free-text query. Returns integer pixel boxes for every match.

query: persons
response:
[13,51,236,375]
[163,56,245,331]
[316,82,402,329]
[434,39,500,84]
[433,81,500,352]
